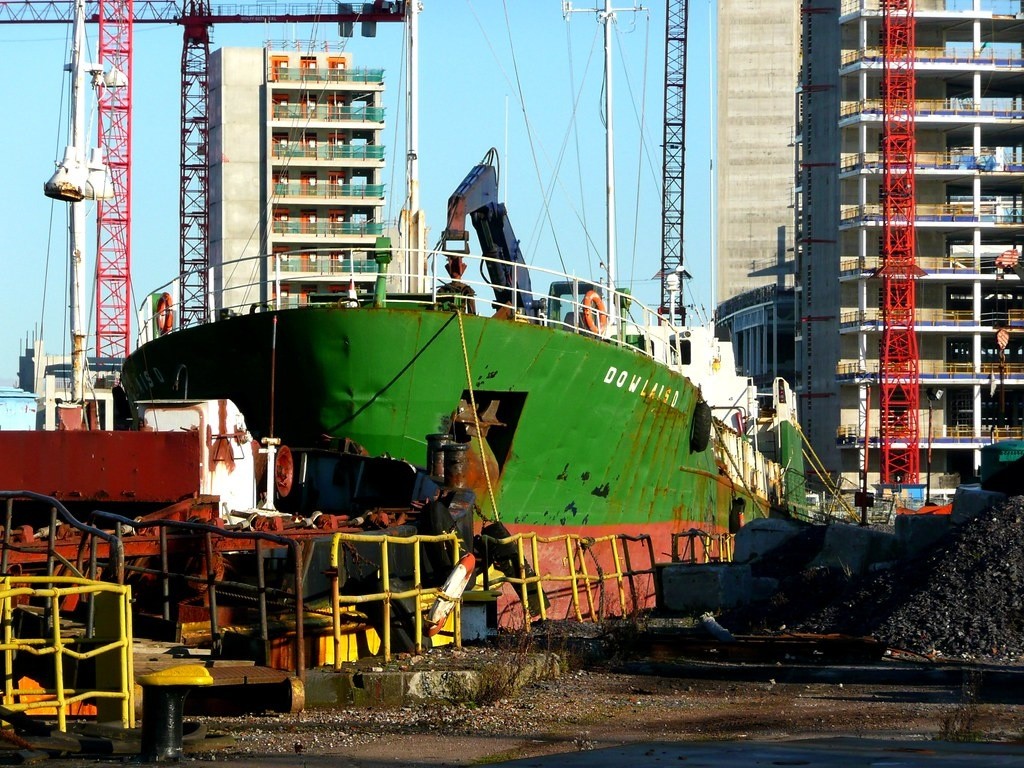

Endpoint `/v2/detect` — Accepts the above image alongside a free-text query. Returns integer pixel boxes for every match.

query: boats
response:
[123,0,860,669]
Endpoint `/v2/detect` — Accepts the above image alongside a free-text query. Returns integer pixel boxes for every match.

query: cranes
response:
[656,0,690,326]
[867,0,927,487]
[0,1,409,396]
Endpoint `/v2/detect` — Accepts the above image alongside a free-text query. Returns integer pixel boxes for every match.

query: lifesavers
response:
[582,289,608,335]
[422,552,477,638]
[689,403,713,453]
[154,290,174,336]
